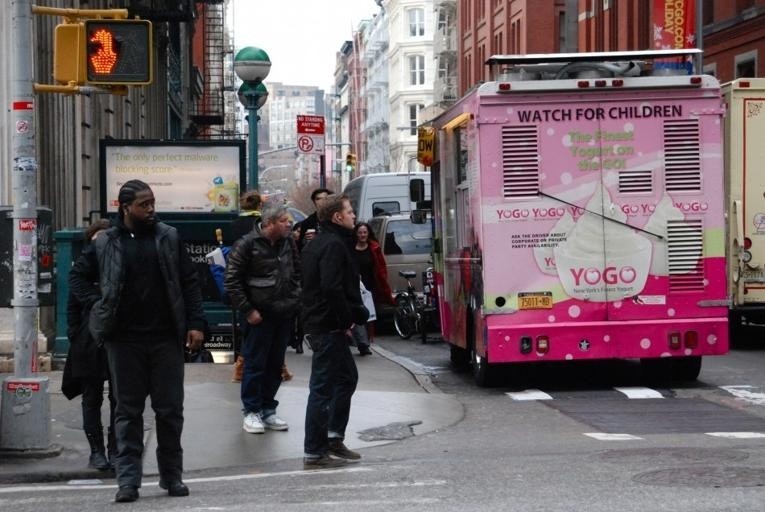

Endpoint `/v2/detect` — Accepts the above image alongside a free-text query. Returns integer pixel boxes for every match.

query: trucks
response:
[717,75,765,344]
[416,44,731,390]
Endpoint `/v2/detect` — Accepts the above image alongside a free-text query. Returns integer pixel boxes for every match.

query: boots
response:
[86,426,110,470]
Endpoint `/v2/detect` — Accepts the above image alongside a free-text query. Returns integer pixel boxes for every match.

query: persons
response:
[291,188,337,253]
[223,200,303,434]
[222,189,294,383]
[67,178,210,504]
[61,217,116,472]
[353,221,400,355]
[299,194,370,470]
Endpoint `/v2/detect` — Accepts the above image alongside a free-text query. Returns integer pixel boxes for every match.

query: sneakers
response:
[116,484,139,502]
[261,414,288,431]
[242,412,266,434]
[158,477,190,496]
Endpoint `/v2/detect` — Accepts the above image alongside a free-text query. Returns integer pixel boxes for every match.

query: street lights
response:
[235,81,269,111]
[259,164,289,182]
[230,43,271,196]
[396,125,418,134]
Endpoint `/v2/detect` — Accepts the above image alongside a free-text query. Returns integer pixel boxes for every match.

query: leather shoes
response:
[304,453,347,469]
[328,439,361,460]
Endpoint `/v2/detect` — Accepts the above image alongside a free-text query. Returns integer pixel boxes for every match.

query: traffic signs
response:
[297,113,327,156]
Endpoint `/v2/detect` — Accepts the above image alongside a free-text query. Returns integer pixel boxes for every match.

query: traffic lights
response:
[75,19,156,92]
[346,153,353,172]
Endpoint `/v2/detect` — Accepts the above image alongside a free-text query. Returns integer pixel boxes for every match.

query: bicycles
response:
[391,271,429,344]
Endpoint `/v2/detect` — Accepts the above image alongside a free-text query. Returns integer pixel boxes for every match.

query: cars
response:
[287,208,318,236]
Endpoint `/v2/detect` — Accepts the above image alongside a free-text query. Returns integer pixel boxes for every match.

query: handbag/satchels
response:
[207,246,234,305]
[360,289,377,323]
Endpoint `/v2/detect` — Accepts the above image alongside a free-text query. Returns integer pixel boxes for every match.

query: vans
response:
[364,211,434,333]
[339,171,433,231]
